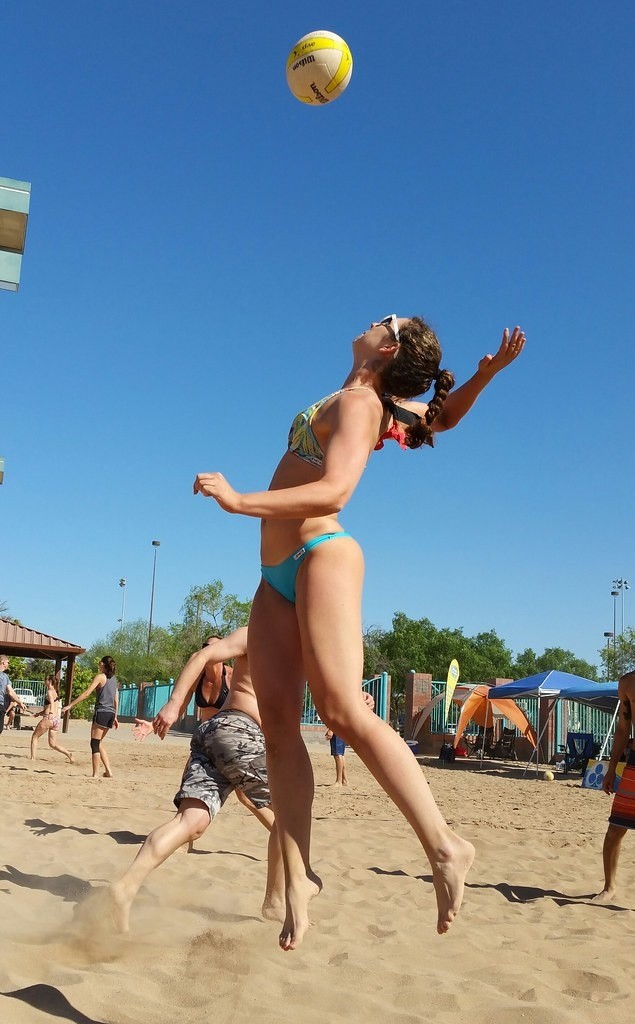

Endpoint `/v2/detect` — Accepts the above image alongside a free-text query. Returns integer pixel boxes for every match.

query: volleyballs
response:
[287,27,355,107]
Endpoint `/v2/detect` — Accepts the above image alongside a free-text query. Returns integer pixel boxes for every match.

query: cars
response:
[14,688,36,707]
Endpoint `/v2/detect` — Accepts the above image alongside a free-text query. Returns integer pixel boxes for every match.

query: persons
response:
[193,311,527,953]
[106,625,375,936]
[4,689,15,730]
[130,635,282,832]
[324,729,350,786]
[29,674,75,764]
[0,654,27,735]
[60,655,119,778]
[589,670,635,901]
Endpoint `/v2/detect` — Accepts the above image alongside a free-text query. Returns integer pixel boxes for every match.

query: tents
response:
[411,682,539,760]
[480,669,621,776]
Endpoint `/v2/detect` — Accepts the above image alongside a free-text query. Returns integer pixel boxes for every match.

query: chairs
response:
[464,726,494,759]
[491,727,517,761]
[563,732,593,777]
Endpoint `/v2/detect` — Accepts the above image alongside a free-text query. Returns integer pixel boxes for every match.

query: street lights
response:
[604,632,614,682]
[611,590,620,682]
[144,540,160,658]
[118,578,126,656]
[612,578,631,674]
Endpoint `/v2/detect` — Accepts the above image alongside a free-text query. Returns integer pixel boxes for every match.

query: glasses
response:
[381,314,400,359]
[1,661,9,664]
[202,643,209,649]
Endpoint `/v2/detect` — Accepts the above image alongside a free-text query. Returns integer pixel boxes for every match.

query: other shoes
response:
[6,725,10,730]
